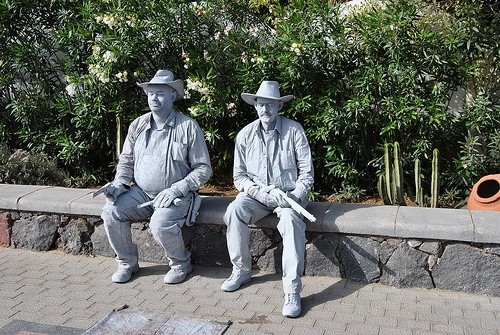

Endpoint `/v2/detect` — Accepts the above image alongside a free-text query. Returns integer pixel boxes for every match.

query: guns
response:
[270,189,317,223]
[92,181,116,198]
[136,197,183,208]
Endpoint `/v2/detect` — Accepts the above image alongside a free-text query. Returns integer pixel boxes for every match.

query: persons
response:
[221,80,314,318]
[101,70,213,285]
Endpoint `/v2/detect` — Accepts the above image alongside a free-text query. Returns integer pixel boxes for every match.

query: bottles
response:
[467,174,500,212]
